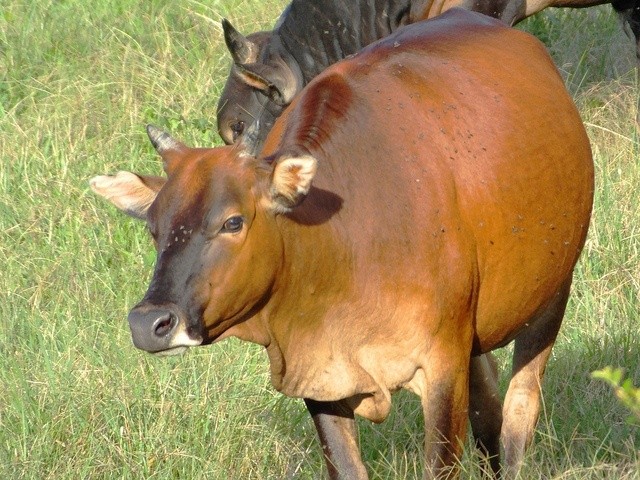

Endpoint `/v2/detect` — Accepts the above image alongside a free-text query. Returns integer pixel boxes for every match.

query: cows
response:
[217,1,639,148]
[89,6,596,480]
